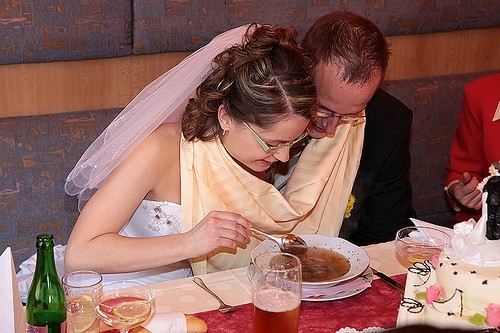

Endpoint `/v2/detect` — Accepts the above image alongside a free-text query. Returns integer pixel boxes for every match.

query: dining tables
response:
[19,222,500,333]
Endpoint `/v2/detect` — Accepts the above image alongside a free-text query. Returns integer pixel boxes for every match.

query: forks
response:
[192,277,243,314]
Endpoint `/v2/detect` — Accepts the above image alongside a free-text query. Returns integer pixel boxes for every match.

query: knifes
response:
[370,266,407,295]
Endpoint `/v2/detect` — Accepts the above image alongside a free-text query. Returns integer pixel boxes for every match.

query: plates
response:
[250,234,370,287]
[246,265,373,302]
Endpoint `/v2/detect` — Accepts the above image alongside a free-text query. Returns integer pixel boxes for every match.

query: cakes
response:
[421,238,500,331]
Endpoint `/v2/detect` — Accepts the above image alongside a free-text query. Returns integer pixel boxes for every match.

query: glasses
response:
[315,110,368,125]
[239,120,311,154]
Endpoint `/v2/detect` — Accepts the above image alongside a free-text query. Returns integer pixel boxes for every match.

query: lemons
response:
[112,301,150,320]
[73,294,97,333]
[407,253,430,264]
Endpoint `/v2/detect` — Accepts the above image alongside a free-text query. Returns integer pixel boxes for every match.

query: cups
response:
[394,226,451,271]
[61,270,103,333]
[251,253,302,333]
[93,281,157,333]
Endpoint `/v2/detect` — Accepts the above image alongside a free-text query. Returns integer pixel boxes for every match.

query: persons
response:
[445,74,500,221]
[275,10,414,246]
[16,24,321,303]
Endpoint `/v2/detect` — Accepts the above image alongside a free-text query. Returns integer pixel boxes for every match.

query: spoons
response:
[245,227,308,256]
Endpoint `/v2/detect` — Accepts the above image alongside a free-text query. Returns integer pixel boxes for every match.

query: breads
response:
[130,313,207,333]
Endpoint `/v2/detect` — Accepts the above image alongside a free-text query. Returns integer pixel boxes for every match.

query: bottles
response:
[25,234,68,333]
